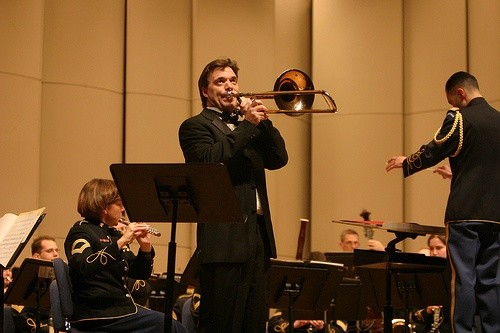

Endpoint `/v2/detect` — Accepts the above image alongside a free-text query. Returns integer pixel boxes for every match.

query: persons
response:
[386,71,500,333]
[3,267,25,314]
[172,287,202,333]
[64,178,185,333]
[31,235,60,261]
[409,234,447,333]
[339,229,385,252]
[179,57,288,333]
[267,308,341,333]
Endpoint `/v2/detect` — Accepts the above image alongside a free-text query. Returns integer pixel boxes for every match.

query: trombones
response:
[229,69,337,113]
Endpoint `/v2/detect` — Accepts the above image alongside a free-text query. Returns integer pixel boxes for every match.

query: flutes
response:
[120,219,161,236]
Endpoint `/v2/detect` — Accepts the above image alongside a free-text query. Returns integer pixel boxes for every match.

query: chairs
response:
[49,258,80,333]
